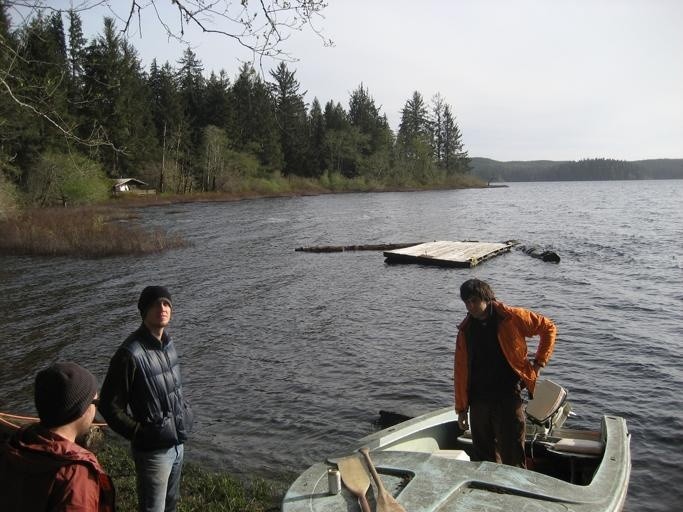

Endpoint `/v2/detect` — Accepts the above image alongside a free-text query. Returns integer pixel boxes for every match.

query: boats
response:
[275,401,636,512]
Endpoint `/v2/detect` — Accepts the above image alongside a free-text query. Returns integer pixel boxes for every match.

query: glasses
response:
[90,396,101,408]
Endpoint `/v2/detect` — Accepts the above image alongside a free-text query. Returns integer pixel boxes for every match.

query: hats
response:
[135,285,173,322]
[33,360,100,431]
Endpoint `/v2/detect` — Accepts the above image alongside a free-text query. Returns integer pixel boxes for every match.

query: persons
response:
[453,279,557,470]
[99,284,187,512]
[0,362,116,512]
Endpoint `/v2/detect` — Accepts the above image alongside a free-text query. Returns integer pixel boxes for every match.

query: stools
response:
[546,436,605,485]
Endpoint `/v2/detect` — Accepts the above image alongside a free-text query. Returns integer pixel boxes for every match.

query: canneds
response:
[327,469,341,495]
